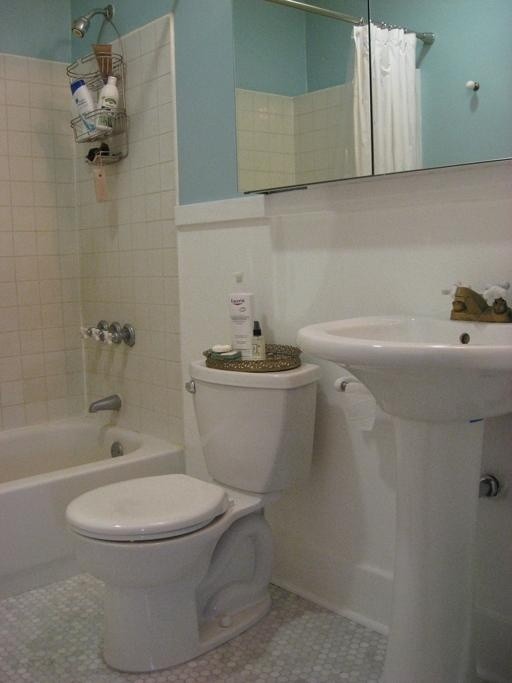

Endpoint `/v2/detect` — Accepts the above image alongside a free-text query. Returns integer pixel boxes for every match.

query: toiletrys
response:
[91,43,112,85]
[253,320,265,360]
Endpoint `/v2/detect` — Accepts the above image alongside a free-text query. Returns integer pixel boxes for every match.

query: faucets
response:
[88,394,121,413]
[449,284,512,323]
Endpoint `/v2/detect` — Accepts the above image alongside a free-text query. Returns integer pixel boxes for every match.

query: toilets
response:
[64,358,320,673]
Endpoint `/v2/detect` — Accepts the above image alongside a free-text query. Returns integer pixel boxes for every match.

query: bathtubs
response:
[0,418,185,600]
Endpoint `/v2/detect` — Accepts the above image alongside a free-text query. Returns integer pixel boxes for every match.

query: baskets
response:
[203,343,302,372]
[66,51,129,164]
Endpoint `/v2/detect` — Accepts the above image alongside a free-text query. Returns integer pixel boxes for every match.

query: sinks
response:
[295,315,512,425]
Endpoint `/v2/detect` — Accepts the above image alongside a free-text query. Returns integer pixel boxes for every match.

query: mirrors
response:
[231,0,512,192]
